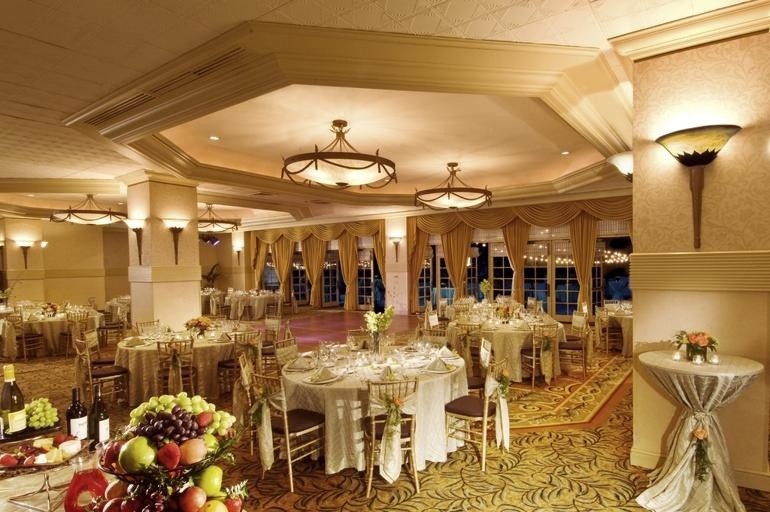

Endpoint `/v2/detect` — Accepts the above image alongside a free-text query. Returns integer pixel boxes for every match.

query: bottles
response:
[64,386,88,444]
[86,384,113,451]
[62,456,111,512]
[0,363,28,436]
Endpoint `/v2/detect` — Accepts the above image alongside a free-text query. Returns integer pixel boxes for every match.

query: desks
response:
[280,344,469,472]
[634,345,767,511]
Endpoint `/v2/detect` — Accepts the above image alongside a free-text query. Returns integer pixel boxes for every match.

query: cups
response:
[708,352,722,365]
[693,354,705,365]
[672,349,681,361]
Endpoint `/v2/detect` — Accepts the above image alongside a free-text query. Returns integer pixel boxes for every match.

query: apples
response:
[0,434,81,465]
[93,434,243,512]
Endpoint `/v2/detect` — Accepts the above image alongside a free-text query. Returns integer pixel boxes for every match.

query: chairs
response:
[444,356,509,474]
[251,373,326,493]
[347,329,375,344]
[419,295,632,389]
[1,285,297,511]
[362,377,420,499]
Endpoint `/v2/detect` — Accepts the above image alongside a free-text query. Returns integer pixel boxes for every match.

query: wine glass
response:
[435,296,560,331]
[0,300,89,323]
[286,328,461,384]
[123,317,258,347]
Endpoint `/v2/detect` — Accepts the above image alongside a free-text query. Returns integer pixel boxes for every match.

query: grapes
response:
[121,392,235,453]
[25,397,58,428]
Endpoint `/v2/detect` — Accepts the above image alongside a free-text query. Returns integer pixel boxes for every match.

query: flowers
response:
[690,423,717,485]
[363,304,396,338]
[669,328,721,362]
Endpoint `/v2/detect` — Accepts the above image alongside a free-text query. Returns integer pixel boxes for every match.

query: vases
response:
[371,334,387,364]
[686,344,710,362]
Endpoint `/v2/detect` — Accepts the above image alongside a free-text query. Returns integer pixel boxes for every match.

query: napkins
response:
[310,366,336,382]
[288,357,311,369]
[378,367,403,381]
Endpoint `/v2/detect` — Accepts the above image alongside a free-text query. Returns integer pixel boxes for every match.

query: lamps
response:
[10,235,39,270]
[162,218,192,266]
[122,217,149,267]
[197,203,243,236]
[413,161,496,215]
[48,194,129,228]
[197,233,222,248]
[605,147,633,186]
[278,118,400,195]
[653,122,745,250]
[231,239,245,268]
